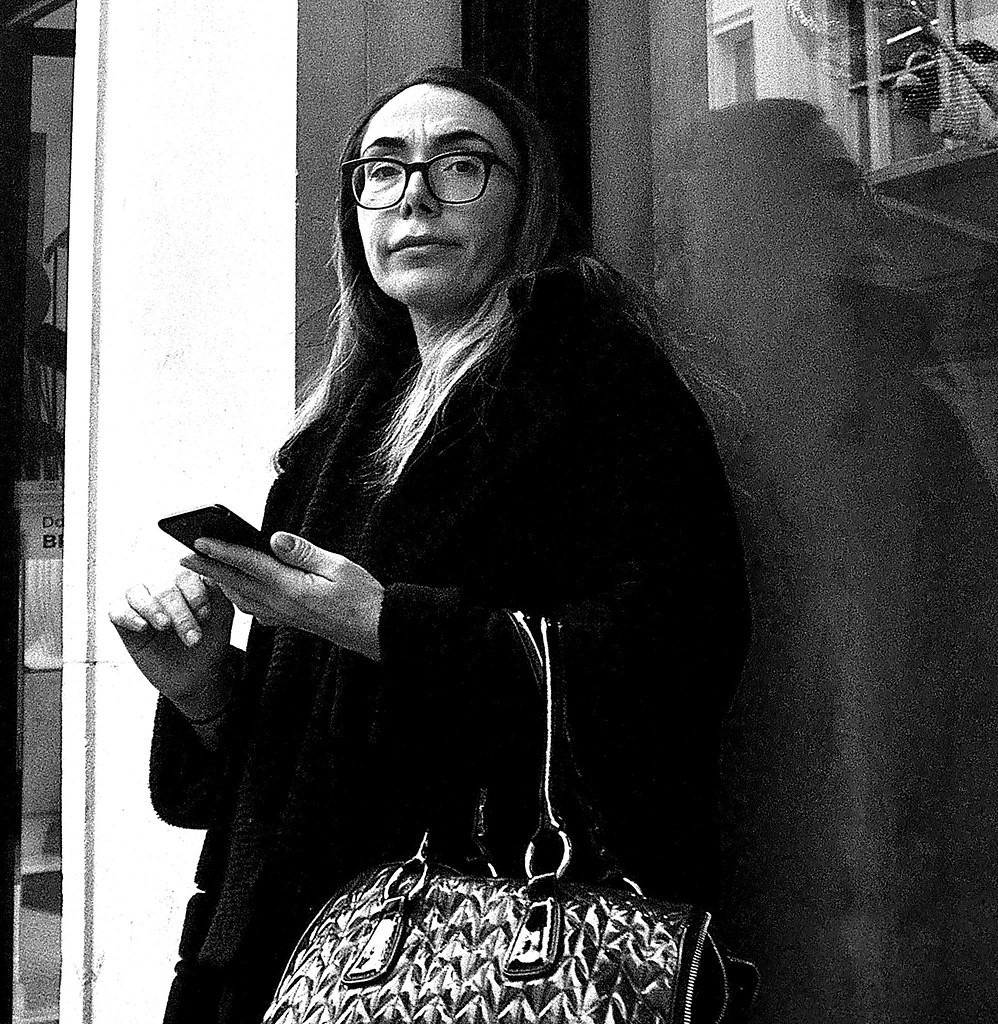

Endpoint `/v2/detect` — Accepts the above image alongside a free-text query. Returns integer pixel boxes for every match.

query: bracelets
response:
[189,705,228,726]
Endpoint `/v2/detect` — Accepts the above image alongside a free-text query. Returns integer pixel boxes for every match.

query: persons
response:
[108,66,759,1024]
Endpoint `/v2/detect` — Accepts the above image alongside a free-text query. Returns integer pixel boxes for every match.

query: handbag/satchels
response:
[259,610,759,1024]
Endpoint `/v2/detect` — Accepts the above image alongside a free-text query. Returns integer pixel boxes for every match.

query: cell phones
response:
[158,505,281,563]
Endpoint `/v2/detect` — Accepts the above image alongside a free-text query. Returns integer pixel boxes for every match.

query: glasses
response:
[807,159,872,200]
[342,151,520,210]
